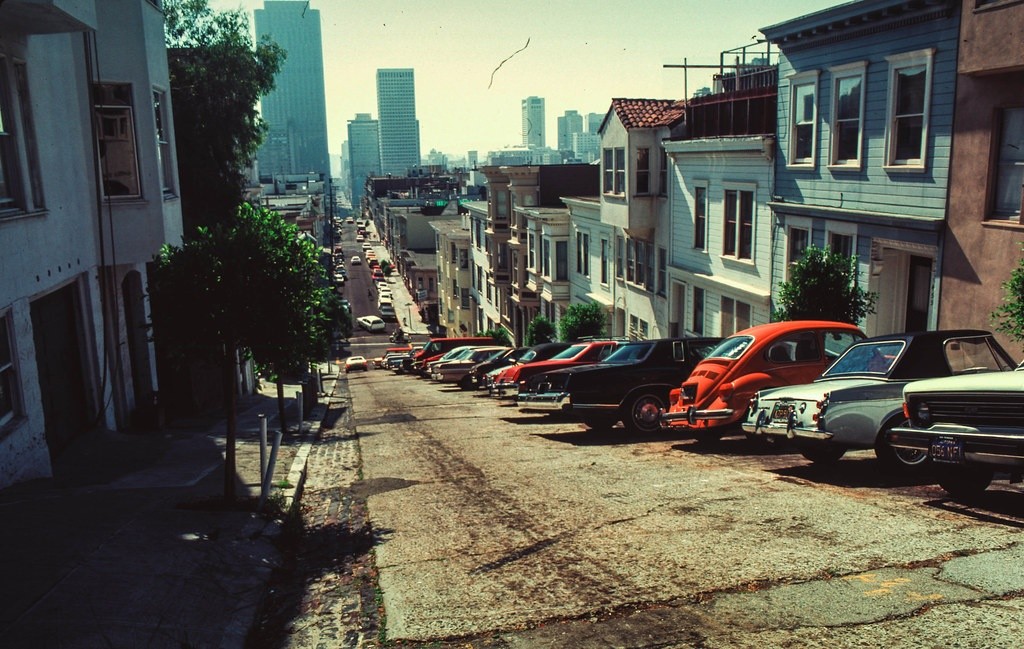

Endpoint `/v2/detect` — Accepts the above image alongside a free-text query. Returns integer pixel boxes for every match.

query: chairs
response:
[795,340,819,359]
[770,346,792,362]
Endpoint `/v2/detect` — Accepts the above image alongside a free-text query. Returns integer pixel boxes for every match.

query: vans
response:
[411,336,495,377]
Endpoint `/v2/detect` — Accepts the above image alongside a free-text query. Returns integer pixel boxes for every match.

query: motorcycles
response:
[390,327,411,343]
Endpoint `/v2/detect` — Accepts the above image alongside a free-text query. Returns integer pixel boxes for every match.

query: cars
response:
[518,335,730,438]
[332,216,396,320]
[658,319,896,448]
[469,343,574,386]
[355,315,386,333]
[344,356,368,373]
[416,346,513,391]
[885,357,1024,497]
[742,327,1018,478]
[372,346,424,375]
[487,339,628,401]
